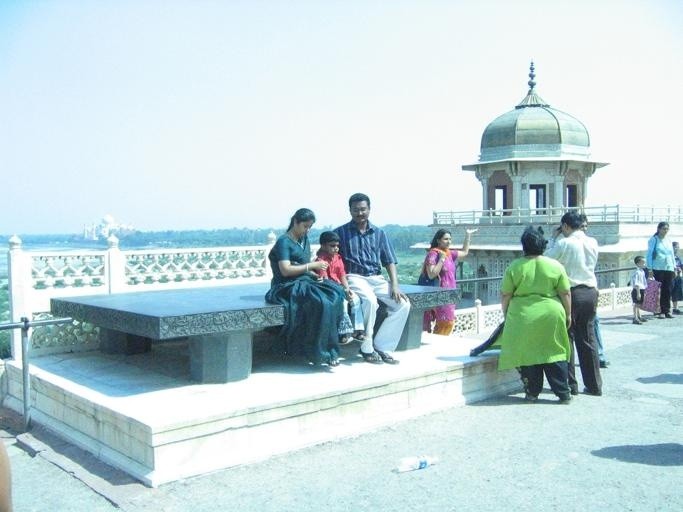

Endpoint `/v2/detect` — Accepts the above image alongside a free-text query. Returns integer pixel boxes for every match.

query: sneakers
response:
[328,350,339,366]
[664,313,672,319]
[338,334,352,343]
[640,317,647,322]
[318,354,330,366]
[600,360,609,369]
[571,390,578,395]
[584,388,602,396]
[354,331,366,341]
[526,394,538,403]
[559,397,570,404]
[655,314,664,319]
[633,318,641,324]
[673,309,681,314]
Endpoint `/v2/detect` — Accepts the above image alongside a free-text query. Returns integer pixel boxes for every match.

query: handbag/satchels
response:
[419,271,442,287]
[641,279,662,312]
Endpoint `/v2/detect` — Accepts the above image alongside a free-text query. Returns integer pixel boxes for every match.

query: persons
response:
[578,214,611,367]
[264,207,346,367]
[468,224,573,405]
[630,256,648,325]
[314,231,367,346]
[332,193,411,366]
[646,221,676,319]
[542,209,603,397]
[669,242,683,315]
[422,227,480,337]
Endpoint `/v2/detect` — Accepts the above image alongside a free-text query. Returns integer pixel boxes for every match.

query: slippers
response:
[375,349,399,364]
[360,349,383,363]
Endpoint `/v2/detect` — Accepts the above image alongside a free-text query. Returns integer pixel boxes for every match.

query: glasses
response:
[325,243,342,248]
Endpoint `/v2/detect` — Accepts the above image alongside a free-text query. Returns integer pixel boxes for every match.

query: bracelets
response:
[306,263,308,273]
[465,239,471,242]
